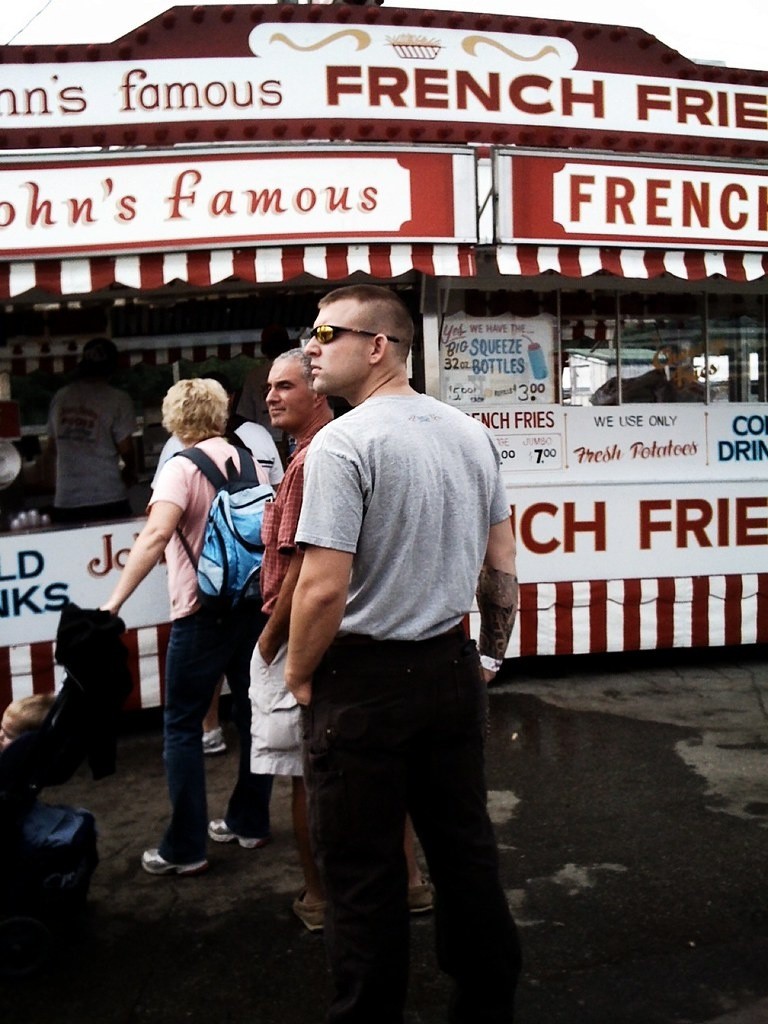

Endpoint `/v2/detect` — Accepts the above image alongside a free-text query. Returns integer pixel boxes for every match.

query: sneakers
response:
[163,728,226,759]
[208,820,266,848]
[141,849,208,876]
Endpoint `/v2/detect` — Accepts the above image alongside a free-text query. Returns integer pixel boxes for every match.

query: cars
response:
[0,729,101,975]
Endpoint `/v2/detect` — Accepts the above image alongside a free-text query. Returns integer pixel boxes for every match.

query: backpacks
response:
[174,444,276,620]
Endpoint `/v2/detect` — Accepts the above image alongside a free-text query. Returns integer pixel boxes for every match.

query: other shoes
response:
[410,879,433,913]
[293,887,326,929]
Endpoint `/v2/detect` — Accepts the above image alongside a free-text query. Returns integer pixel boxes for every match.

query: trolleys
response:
[0,594,133,792]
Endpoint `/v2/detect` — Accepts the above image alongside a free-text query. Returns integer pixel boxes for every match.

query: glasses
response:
[310,325,400,343]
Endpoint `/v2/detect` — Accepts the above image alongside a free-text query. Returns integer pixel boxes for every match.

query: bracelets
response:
[478,656,502,673]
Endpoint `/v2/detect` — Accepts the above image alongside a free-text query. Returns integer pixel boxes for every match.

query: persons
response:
[0,689,60,757]
[235,324,290,469]
[152,371,284,758]
[282,282,519,1024]
[244,348,435,932]
[94,377,276,882]
[43,337,142,525]
